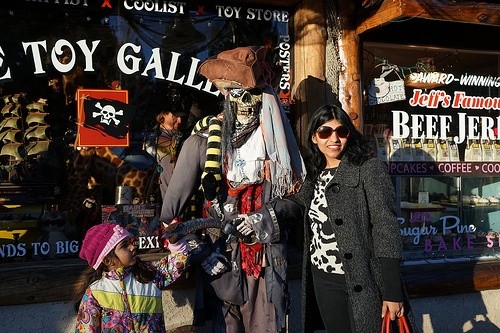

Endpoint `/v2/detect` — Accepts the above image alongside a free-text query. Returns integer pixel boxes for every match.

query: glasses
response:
[314,125,349,139]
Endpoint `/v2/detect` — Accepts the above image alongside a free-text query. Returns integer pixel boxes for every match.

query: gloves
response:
[236,214,255,236]
[193,245,230,277]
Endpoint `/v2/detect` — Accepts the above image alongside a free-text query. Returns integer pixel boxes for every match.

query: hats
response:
[79,223,130,270]
[197,45,272,90]
[152,96,187,118]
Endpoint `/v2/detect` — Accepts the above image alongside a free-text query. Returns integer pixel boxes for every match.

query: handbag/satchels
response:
[210,269,245,306]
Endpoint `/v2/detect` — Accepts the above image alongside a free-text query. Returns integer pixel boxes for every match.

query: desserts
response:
[448,194,500,206]
[466,226,500,242]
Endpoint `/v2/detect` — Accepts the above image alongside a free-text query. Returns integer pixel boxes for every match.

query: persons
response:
[299,102,405,333]
[73,217,191,333]
[143,98,191,217]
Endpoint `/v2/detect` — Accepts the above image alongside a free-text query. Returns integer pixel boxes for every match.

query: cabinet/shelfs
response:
[389,175,500,266]
